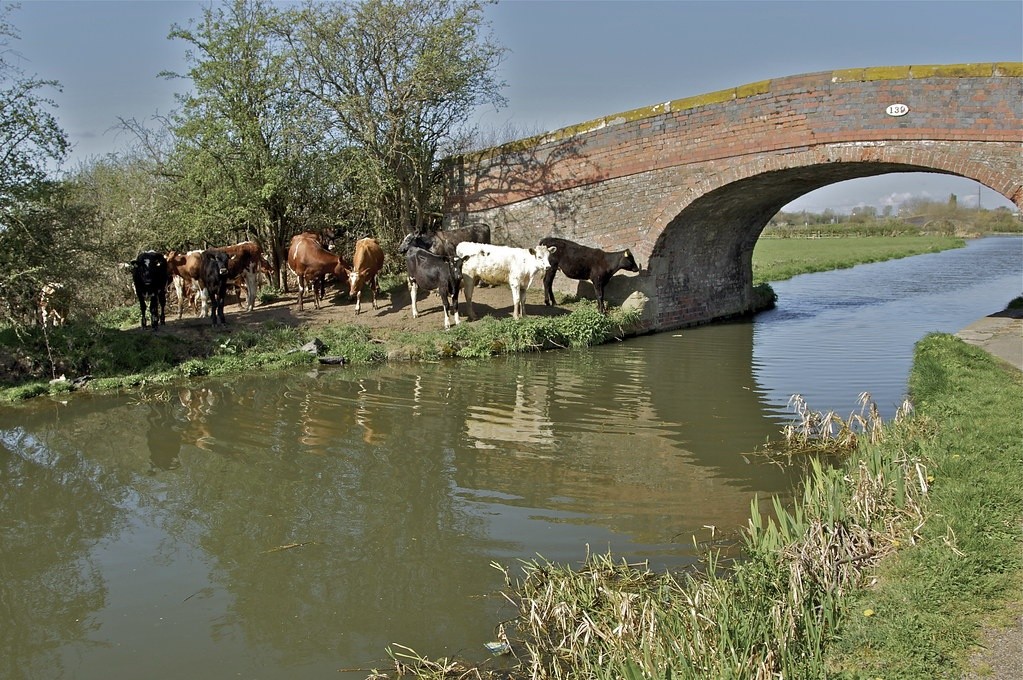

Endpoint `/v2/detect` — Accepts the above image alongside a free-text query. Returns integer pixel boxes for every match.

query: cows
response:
[124,249,170,336]
[161,239,267,327]
[395,224,491,327]
[454,241,554,321]
[288,233,383,318]
[539,238,640,315]
